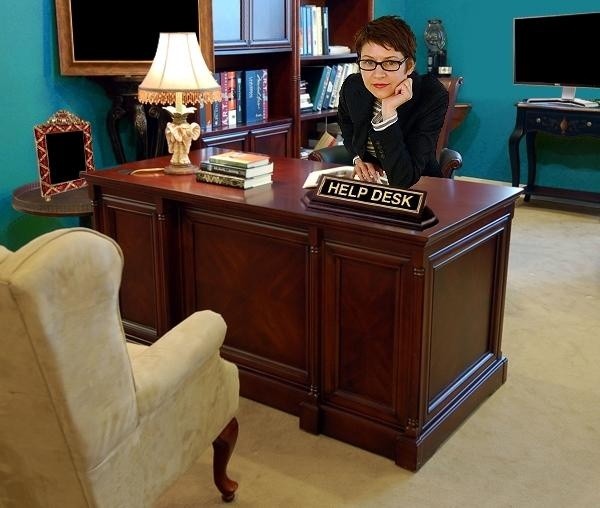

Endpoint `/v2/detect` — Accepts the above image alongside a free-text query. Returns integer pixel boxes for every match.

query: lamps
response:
[139,31,223,176]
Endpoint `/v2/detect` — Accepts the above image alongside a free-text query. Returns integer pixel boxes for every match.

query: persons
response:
[335,13,449,190]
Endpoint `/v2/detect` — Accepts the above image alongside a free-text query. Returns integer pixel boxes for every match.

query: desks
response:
[13,179,94,227]
[79,151,524,473]
[508,100,600,204]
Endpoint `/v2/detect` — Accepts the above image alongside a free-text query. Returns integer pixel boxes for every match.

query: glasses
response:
[357,54,409,72]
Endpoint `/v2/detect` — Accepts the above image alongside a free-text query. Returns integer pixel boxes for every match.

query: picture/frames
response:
[52,0,216,76]
[34,112,95,203]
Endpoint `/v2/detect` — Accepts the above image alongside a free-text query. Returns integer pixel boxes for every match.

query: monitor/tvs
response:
[513,12,600,108]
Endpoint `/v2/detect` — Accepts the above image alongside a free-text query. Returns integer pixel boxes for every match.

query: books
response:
[194,4,360,190]
[301,163,391,191]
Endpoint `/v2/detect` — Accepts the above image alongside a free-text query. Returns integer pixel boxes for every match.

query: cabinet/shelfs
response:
[193,0,375,158]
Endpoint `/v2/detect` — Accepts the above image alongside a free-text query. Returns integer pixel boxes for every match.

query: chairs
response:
[308,76,462,179]
[1,226,240,507]
